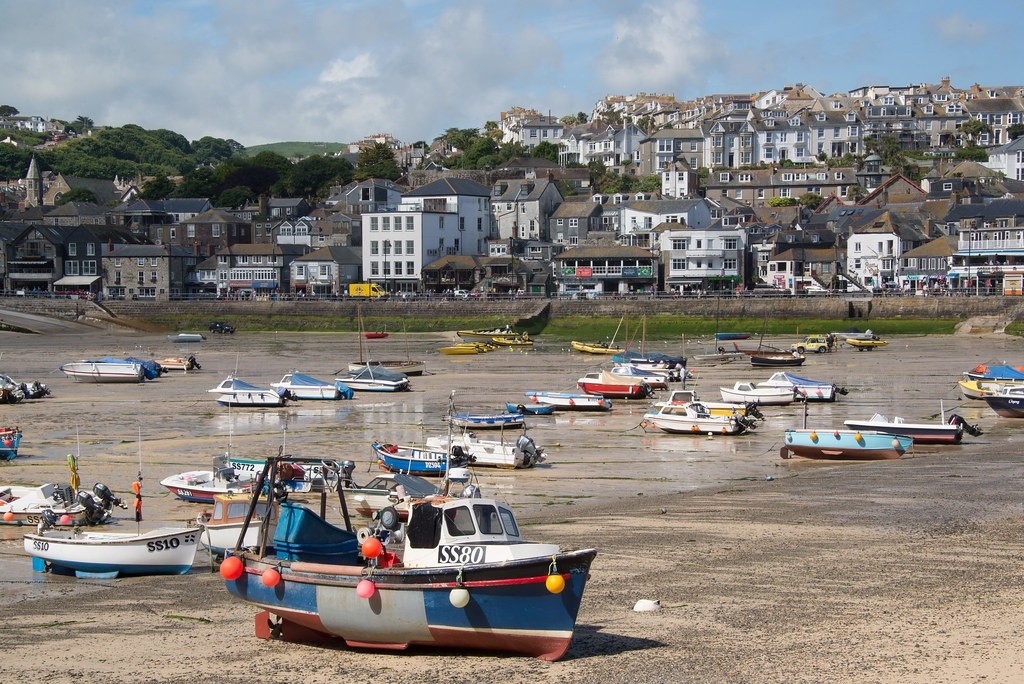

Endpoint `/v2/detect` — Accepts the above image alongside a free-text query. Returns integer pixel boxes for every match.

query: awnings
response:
[953,247,1024,257]
[949,273,958,278]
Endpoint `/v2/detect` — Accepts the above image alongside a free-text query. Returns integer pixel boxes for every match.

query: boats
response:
[1,311,1024,576]
[221,389,598,662]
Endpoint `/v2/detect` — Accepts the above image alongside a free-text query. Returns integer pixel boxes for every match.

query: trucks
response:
[348,282,389,301]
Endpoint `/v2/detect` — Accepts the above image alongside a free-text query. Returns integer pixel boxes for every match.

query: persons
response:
[425,288,520,303]
[236,289,306,301]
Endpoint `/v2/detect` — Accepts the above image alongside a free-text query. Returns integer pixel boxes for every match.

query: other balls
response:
[691,426,699,430]
[642,423,648,427]
[448,588,470,608]
[261,568,280,587]
[219,556,244,581]
[689,368,695,373]
[568,400,574,405]
[815,389,822,395]
[355,579,375,599]
[854,434,862,442]
[2,512,14,522]
[4,440,12,447]
[650,423,656,428]
[722,428,727,433]
[892,440,900,448]
[598,400,603,405]
[978,391,984,396]
[60,514,70,523]
[545,573,565,594]
[833,432,839,437]
[530,396,537,401]
[377,459,383,464]
[786,435,793,440]
[361,538,382,558]
[809,433,817,441]
[799,388,806,395]
